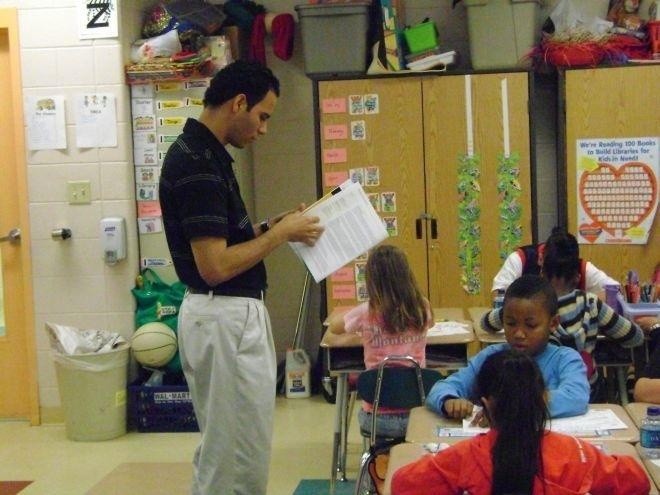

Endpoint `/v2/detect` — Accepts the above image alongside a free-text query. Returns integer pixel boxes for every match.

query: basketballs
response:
[130,322,177,366]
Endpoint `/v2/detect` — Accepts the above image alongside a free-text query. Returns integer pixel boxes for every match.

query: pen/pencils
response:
[625,281,655,303]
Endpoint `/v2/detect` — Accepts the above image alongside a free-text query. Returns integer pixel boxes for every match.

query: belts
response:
[188,280,265,300]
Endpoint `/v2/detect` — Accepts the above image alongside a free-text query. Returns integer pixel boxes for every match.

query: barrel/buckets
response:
[284,347,312,399]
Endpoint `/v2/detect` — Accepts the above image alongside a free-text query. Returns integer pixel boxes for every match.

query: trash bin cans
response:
[49,340,131,442]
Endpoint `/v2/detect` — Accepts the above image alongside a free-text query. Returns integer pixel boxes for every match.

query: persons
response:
[157,60,326,495]
[489,227,621,296]
[424,275,591,429]
[330,245,436,467]
[390,349,651,495]
[480,248,646,405]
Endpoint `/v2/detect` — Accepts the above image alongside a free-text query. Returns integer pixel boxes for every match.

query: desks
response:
[385,442,660,495]
[626,400,660,430]
[323,306,465,328]
[636,438,660,492]
[468,303,634,404]
[402,403,645,445]
[321,325,478,463]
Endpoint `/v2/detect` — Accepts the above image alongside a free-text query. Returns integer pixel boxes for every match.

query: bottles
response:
[640,406,660,460]
[495,288,505,333]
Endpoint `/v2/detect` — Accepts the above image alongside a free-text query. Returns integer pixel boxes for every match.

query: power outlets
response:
[68,180,90,204]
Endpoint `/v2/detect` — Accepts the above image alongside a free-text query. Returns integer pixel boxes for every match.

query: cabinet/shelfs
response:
[559,64,660,306]
[309,76,535,317]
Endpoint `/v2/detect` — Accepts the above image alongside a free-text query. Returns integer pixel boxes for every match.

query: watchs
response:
[260,221,269,232]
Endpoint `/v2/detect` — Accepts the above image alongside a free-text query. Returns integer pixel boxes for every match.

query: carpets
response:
[293,475,364,495]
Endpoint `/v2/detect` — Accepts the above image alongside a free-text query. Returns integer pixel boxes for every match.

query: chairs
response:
[361,351,423,461]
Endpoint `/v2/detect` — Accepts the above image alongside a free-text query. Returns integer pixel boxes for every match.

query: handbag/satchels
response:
[131,268,188,377]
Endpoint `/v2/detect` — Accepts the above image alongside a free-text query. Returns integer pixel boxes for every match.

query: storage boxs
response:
[465,0,545,73]
[297,2,370,76]
